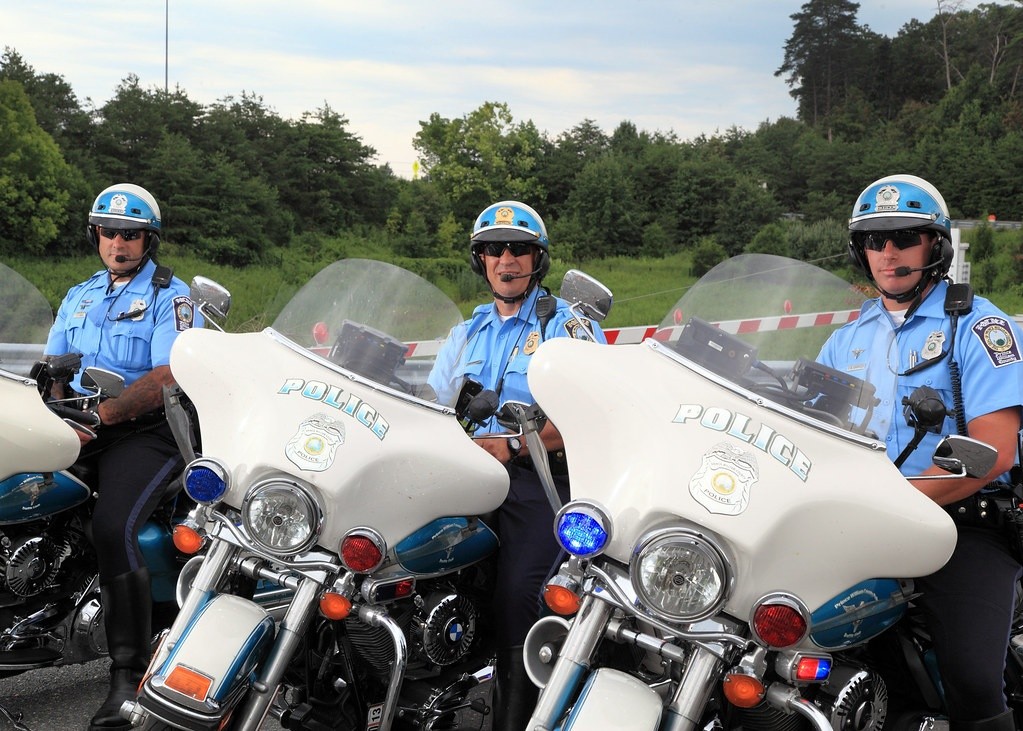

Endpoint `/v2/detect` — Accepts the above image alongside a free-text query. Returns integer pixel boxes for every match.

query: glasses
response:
[98,225,145,241]
[478,241,533,257]
[858,227,933,252]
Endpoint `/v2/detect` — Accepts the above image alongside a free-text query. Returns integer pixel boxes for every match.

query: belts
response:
[66,388,164,421]
[938,495,996,526]
[509,452,568,476]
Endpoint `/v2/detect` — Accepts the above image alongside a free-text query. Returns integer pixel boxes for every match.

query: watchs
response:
[86,406,109,431]
[502,431,522,461]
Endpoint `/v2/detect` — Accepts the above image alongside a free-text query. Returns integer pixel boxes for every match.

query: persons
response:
[803,174,1023,731]
[427,200,608,731]
[39,181,206,731]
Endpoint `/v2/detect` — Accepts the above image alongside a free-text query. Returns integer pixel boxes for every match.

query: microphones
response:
[895,257,946,277]
[115,248,151,262]
[500,267,543,283]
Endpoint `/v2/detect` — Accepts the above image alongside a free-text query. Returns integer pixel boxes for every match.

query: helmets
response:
[88,183,162,258]
[847,174,954,278]
[469,200,550,277]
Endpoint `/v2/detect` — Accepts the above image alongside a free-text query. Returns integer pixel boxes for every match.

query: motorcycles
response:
[112,256,615,731]
[523,251,1023,731]
[0,258,204,731]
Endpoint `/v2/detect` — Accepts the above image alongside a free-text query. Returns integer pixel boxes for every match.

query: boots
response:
[492,643,535,731]
[86,566,152,731]
[947,707,1018,731]
